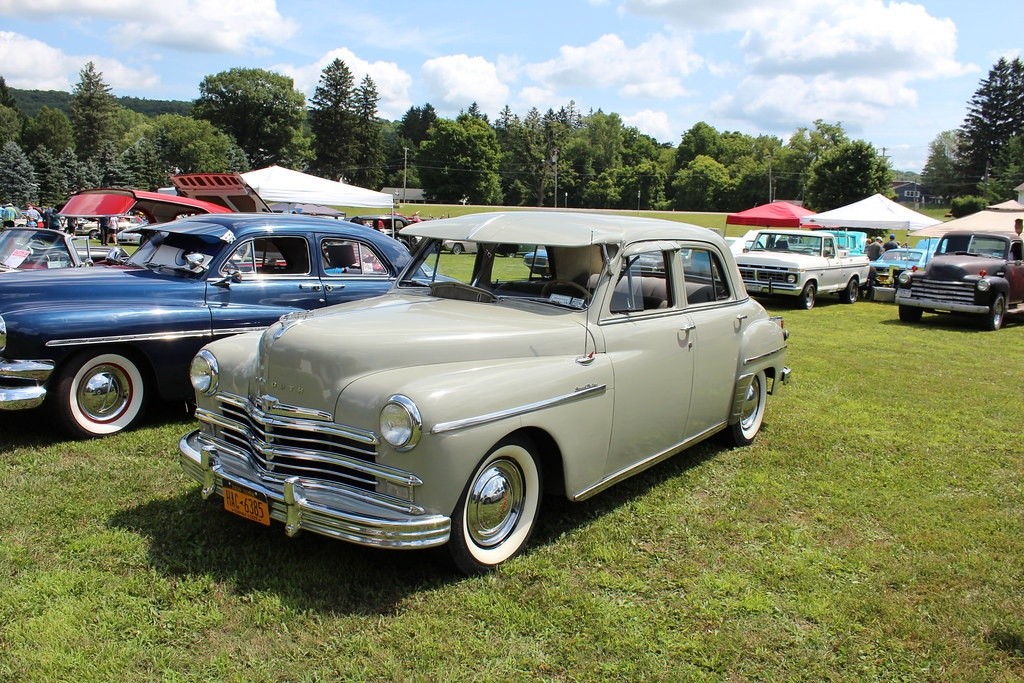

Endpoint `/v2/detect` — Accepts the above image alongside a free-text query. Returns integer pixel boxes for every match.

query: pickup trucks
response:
[735,230,870,310]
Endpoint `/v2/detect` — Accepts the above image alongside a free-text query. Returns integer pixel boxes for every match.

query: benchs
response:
[495,279,667,314]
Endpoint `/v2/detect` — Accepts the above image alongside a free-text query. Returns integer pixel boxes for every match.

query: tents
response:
[158,166,396,239]
[907,200,1024,269]
[722,201,839,239]
[263,203,346,220]
[796,192,942,248]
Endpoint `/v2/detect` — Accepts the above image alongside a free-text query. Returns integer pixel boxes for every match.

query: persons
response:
[0,202,78,236]
[2,203,17,228]
[864,238,872,253]
[896,241,901,248]
[866,237,882,261]
[882,234,898,260]
[97,216,119,246]
[49,208,63,230]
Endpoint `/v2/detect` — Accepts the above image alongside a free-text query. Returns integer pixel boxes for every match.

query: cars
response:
[82,216,148,246]
[442,240,477,254]
[0,188,234,279]
[522,249,552,276]
[179,211,792,569]
[0,214,464,437]
[870,238,947,288]
[895,231,1024,330]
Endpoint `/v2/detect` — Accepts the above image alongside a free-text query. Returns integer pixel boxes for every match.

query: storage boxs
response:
[872,285,896,302]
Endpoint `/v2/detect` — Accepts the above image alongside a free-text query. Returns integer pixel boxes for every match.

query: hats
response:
[6,202,12,206]
[890,234,895,239]
[875,236,883,242]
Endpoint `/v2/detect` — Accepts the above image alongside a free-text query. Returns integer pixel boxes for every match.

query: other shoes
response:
[115,243,118,246]
[106,243,109,245]
[102,243,105,246]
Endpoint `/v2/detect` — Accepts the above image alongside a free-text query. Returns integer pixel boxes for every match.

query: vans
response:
[343,215,411,252]
